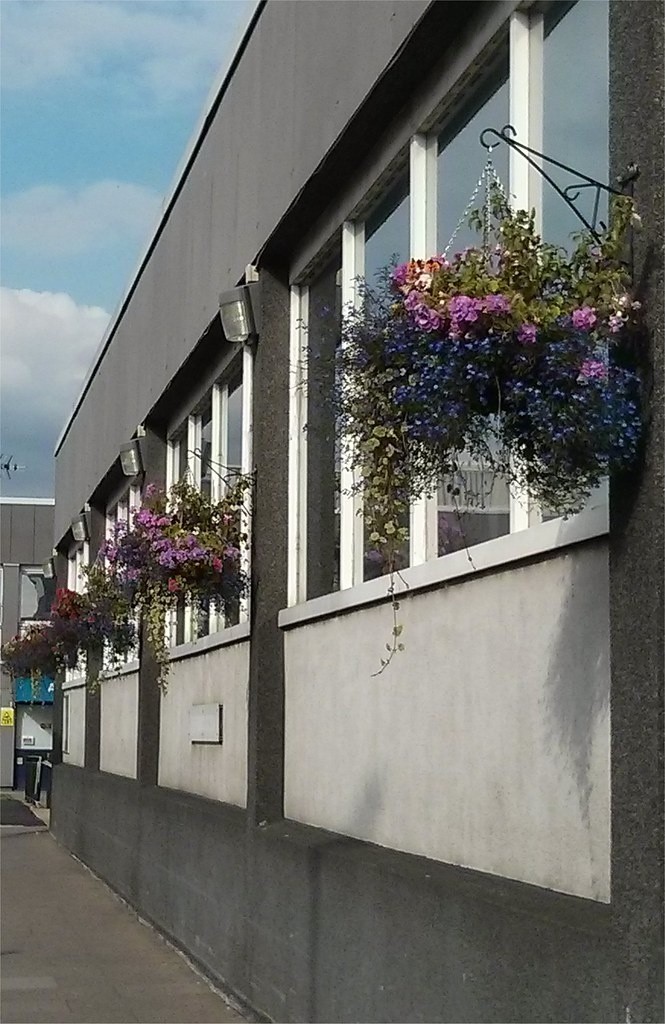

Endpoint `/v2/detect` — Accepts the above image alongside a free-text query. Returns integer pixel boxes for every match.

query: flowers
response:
[2,472,260,697]
[298,194,645,594]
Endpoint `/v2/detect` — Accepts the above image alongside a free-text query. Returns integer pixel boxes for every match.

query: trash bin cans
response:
[25,754,44,802]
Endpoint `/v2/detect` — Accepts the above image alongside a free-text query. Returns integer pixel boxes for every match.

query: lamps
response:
[71,514,86,541]
[219,285,253,347]
[118,439,142,476]
[40,557,54,579]
